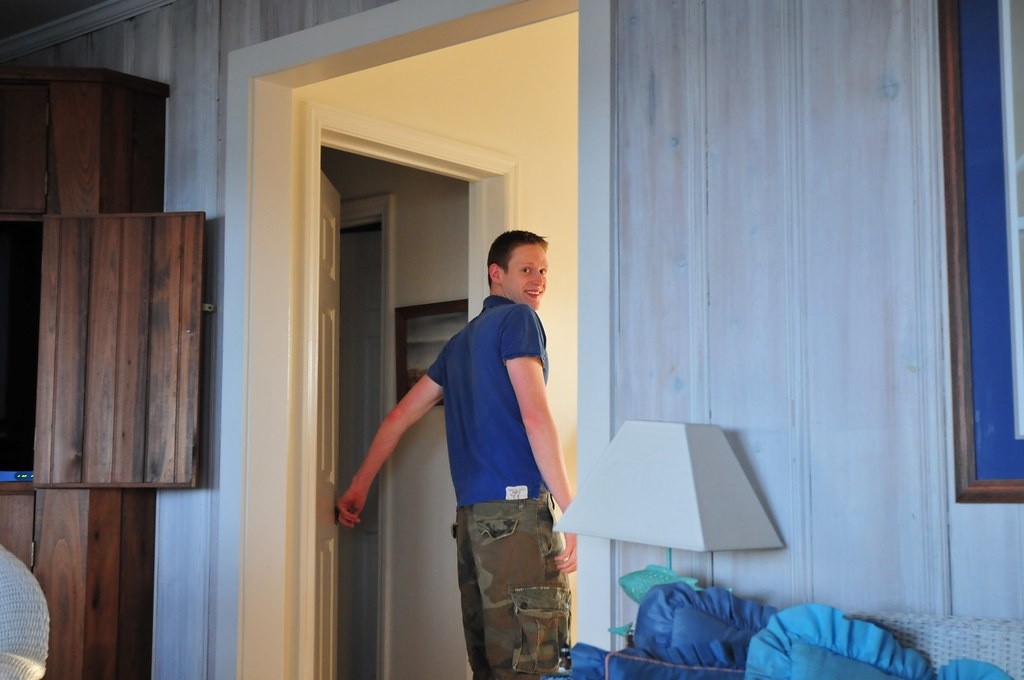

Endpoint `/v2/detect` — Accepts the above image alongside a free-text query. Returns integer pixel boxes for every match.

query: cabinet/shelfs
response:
[0,64,216,680]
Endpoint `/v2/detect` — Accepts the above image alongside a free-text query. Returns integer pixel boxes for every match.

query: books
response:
[0,470,34,481]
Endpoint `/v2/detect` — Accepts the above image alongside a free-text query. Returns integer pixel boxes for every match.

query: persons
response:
[335,231,577,680]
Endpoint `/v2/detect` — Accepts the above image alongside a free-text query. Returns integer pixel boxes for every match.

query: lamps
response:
[555,420,788,645]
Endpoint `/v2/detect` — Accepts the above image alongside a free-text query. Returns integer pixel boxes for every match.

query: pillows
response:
[532,582,1014,680]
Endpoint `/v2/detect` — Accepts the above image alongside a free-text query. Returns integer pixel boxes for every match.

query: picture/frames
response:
[937,0,1024,504]
[394,299,469,405]
